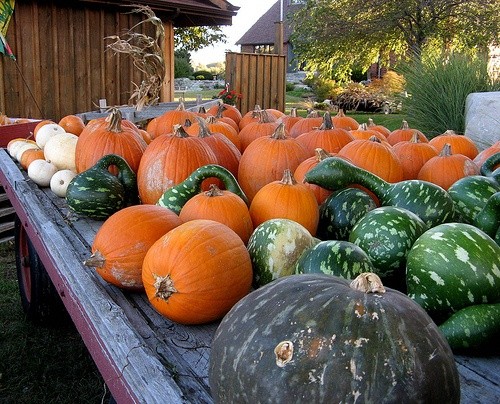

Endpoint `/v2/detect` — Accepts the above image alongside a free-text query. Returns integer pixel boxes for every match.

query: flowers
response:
[211,89,243,106]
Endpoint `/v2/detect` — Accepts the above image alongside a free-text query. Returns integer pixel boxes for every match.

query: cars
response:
[286,70,321,86]
[174,77,226,90]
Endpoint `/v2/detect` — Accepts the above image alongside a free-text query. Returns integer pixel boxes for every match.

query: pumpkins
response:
[0,102,500,404]
